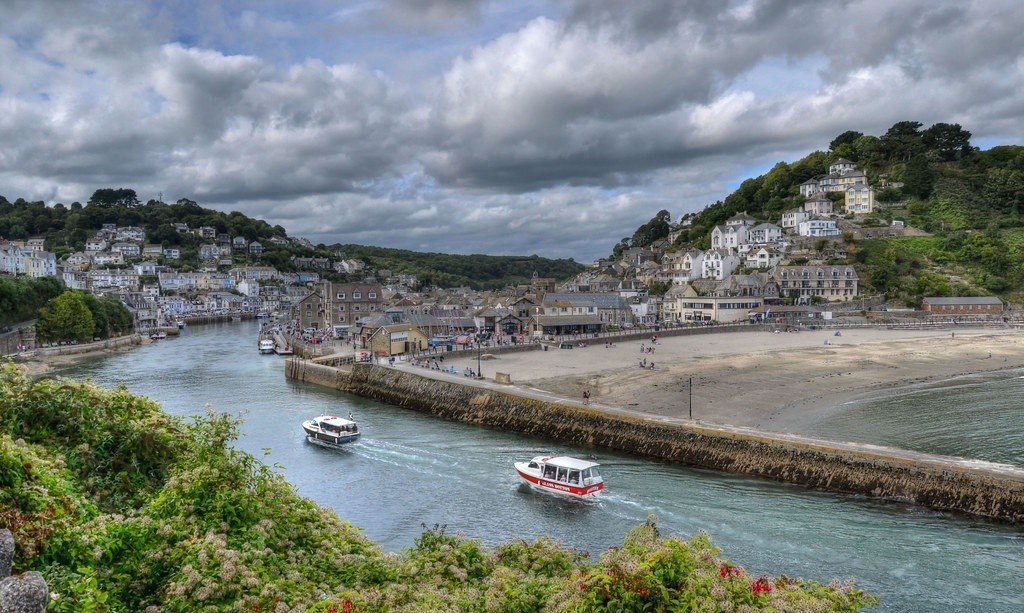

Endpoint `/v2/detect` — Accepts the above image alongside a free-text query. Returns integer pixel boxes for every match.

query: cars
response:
[461,329,478,338]
[623,323,634,330]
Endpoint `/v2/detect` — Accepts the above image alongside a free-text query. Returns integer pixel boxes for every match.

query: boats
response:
[257,312,268,319]
[259,338,276,355]
[303,414,360,446]
[178,321,185,329]
[151,333,167,340]
[514,453,606,501]
[270,311,285,320]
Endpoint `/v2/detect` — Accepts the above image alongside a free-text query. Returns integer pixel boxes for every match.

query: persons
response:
[526,329,659,370]
[582,390,590,405]
[544,470,578,486]
[278,310,524,379]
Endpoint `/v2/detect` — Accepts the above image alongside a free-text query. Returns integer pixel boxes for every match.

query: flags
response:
[348,413,354,421]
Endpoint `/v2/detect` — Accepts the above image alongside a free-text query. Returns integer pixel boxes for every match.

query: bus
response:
[334,325,352,340]
